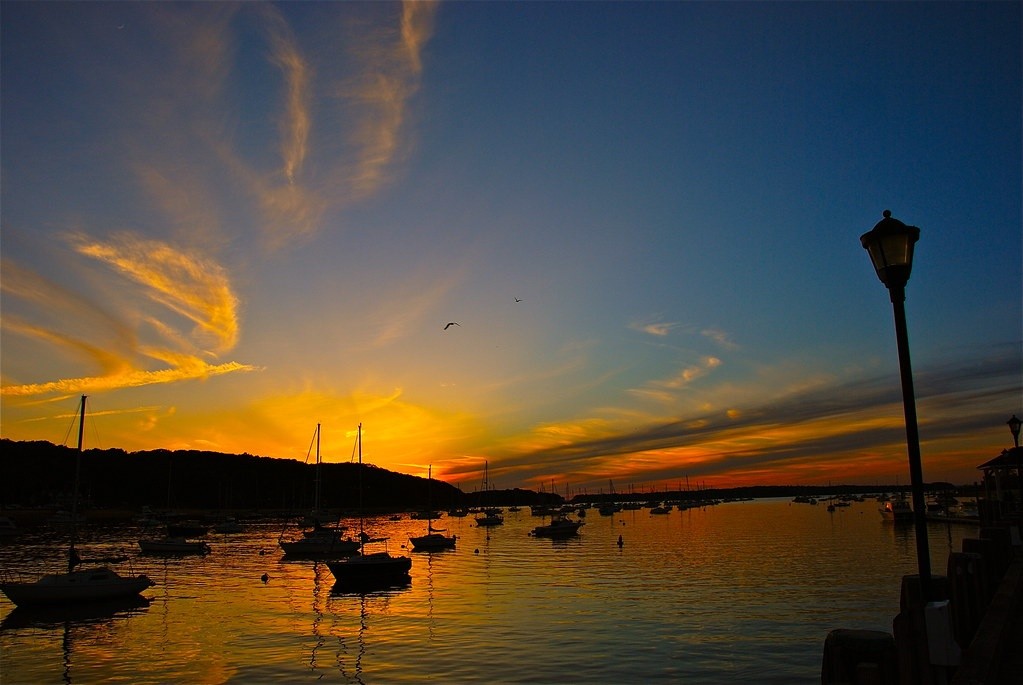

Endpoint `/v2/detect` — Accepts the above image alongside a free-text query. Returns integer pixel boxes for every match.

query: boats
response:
[958,501,980,519]
[792,493,896,511]
[159,510,263,538]
[925,501,948,522]
[934,488,959,506]
[879,500,914,522]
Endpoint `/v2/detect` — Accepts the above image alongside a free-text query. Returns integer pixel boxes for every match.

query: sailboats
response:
[408,465,457,549]
[137,462,211,553]
[0,394,158,612]
[530,479,586,537]
[410,460,754,527]
[278,423,369,555]
[324,423,412,582]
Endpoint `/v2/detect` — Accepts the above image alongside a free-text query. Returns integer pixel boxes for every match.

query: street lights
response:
[859,206,949,685]
[1006,414,1023,447]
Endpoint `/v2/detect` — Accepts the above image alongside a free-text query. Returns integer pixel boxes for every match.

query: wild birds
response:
[514,297,523,302]
[444,322,460,330]
